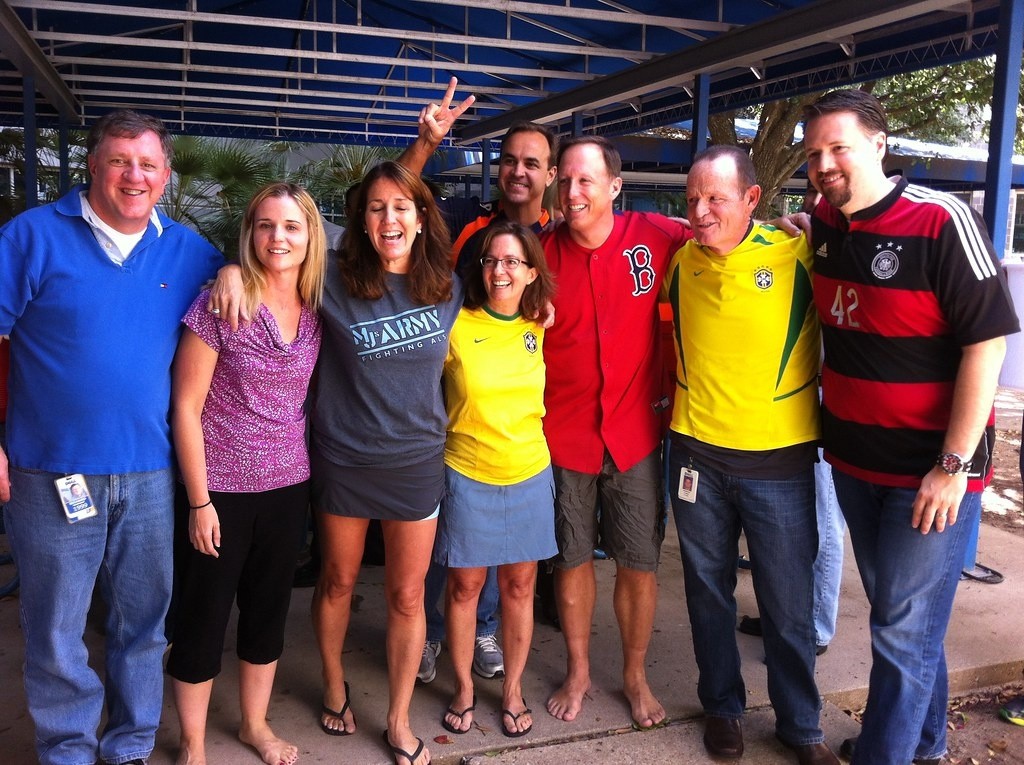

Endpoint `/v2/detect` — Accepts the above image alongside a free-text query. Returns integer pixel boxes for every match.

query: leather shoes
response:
[841,737,941,765]
[776,730,841,765]
[704,716,744,758]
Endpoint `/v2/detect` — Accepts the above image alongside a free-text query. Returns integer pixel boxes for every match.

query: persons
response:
[666,91,1022,765]
[684,478,692,489]
[740,182,848,664]
[165,184,327,765]
[661,144,841,765]
[70,484,82,497]
[544,135,816,727]
[206,161,556,765]
[397,75,565,684]
[434,222,557,736]
[0,112,225,765]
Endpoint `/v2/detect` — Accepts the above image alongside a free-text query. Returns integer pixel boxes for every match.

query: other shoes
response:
[738,556,752,569]
[119,758,148,765]
[740,617,762,636]
[291,558,321,588]
[817,645,827,655]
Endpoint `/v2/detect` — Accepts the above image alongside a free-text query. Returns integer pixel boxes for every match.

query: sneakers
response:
[999,696,1024,727]
[473,636,504,678]
[415,641,441,683]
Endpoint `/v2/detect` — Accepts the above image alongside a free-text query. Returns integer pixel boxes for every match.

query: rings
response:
[212,309,219,313]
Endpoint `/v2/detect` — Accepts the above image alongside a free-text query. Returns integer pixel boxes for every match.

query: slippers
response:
[443,695,477,734]
[383,729,431,765]
[320,680,356,736]
[502,697,532,738]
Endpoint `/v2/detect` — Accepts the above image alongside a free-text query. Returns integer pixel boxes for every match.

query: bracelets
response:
[190,500,212,509]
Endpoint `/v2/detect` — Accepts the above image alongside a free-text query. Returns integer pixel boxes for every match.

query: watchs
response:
[935,453,973,475]
